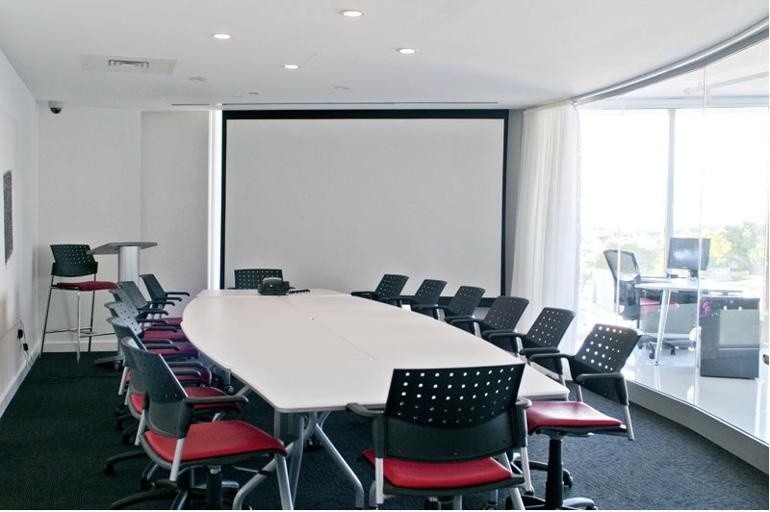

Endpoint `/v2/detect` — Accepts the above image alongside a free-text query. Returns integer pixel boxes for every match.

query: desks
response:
[634,275,744,366]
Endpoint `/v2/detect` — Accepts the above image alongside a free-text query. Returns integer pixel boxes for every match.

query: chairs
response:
[38,244,125,363]
[603,248,677,358]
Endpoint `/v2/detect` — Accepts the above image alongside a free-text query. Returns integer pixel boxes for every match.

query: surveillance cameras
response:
[48,100,63,114]
